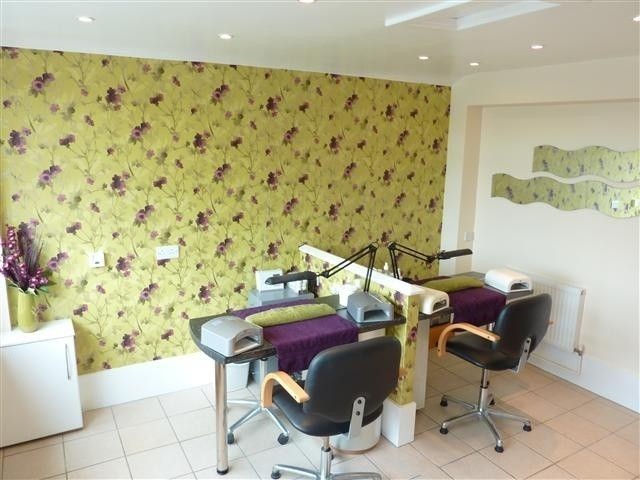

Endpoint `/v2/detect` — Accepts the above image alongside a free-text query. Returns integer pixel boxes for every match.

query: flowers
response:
[0,219,56,322]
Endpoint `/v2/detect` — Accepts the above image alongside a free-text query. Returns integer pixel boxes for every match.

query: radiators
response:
[535,274,585,354]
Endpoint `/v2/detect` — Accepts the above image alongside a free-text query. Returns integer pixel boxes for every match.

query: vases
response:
[17,292,40,333]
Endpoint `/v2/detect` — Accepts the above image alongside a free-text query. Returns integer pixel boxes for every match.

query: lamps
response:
[388,241,472,279]
[265,239,379,292]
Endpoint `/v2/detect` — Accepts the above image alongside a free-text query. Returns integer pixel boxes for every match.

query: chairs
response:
[260,335,401,480]
[435,293,552,452]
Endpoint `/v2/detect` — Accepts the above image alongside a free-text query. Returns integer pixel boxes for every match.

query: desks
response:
[415,271,534,410]
[188,295,406,475]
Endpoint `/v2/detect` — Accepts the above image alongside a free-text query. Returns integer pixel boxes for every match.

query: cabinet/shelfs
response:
[0,318,84,448]
[249,286,314,385]
